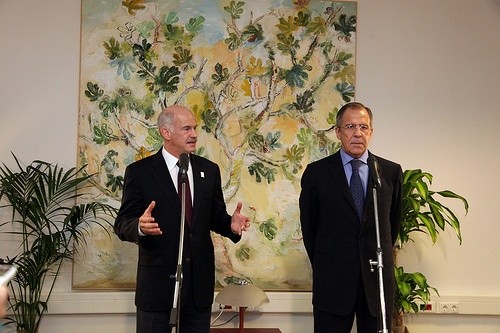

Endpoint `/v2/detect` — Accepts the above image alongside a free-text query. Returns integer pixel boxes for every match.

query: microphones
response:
[179,153,189,184]
[367,157,381,187]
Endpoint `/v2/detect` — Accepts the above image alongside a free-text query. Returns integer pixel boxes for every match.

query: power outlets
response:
[219,304,232,309]
[439,302,459,314]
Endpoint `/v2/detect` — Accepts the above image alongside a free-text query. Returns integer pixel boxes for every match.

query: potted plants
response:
[391,168,469,333]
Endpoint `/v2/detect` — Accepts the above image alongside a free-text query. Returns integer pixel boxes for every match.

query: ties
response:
[176,159,194,231]
[349,160,365,226]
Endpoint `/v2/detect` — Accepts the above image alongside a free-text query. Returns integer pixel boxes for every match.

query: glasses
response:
[336,124,373,131]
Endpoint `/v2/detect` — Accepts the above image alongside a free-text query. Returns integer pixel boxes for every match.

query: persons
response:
[113,105,250,333]
[298,102,405,333]
